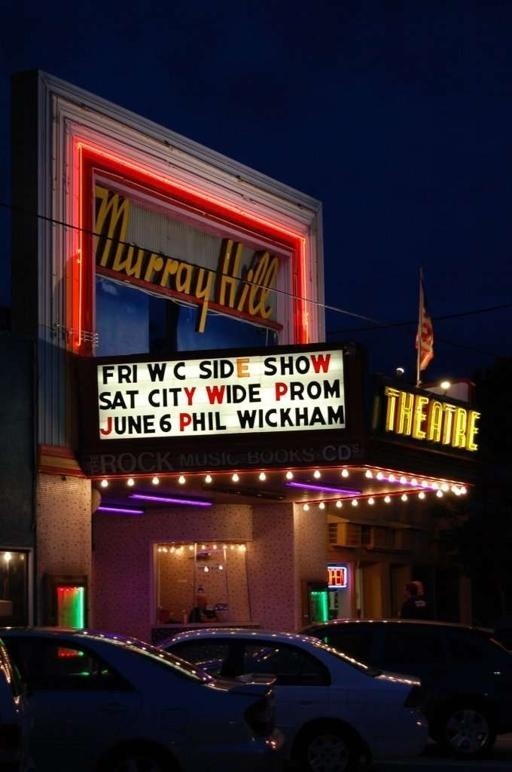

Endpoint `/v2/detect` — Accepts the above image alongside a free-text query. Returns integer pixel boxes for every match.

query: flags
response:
[413,276,434,372]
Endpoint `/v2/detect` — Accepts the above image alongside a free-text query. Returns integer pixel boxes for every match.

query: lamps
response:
[440,379,451,397]
[98,467,467,512]
[394,366,406,384]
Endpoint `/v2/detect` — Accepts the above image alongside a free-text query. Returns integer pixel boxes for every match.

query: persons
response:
[396,579,429,621]
